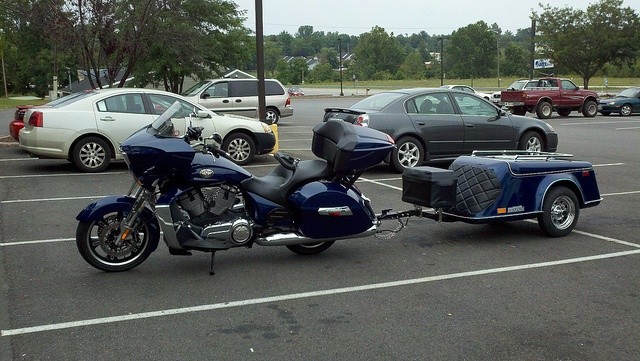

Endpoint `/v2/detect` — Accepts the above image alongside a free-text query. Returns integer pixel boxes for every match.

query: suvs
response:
[491,79,548,114]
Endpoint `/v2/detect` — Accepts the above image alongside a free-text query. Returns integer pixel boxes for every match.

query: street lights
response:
[487,29,500,88]
[439,37,443,86]
[338,39,344,96]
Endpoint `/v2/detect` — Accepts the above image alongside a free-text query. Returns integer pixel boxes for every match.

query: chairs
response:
[135,104,141,112]
[420,100,434,113]
[438,101,452,113]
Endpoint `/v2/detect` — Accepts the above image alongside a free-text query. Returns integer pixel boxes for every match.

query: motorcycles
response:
[75,101,397,275]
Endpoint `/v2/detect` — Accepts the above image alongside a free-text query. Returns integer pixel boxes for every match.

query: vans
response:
[179,78,293,126]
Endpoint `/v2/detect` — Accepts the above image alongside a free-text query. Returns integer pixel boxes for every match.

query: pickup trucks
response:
[502,77,599,119]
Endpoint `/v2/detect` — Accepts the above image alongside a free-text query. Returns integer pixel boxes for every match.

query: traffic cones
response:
[270,124,278,154]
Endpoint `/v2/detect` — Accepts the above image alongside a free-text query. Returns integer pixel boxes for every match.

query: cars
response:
[18,88,276,173]
[424,85,491,109]
[288,86,304,97]
[324,87,558,173]
[10,90,91,142]
[597,87,640,116]
